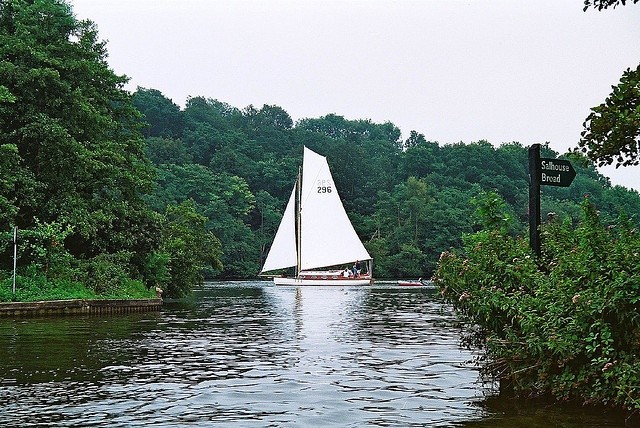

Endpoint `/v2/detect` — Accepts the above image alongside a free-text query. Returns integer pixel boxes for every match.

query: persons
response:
[344,268,350,277]
[356,260,361,278]
[353,262,358,277]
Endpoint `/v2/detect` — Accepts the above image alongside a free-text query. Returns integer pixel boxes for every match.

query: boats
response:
[397,278,426,286]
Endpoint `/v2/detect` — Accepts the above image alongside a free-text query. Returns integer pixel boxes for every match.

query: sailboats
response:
[256,142,374,286]
